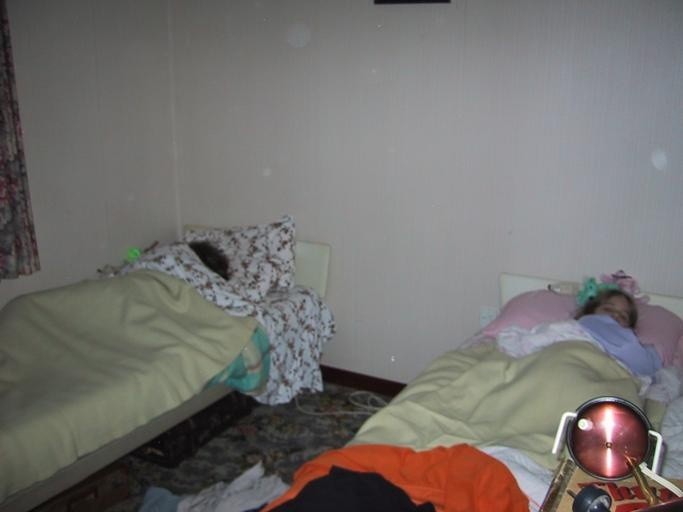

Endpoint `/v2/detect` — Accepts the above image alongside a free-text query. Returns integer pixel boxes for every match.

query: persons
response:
[575,290,637,329]
[188,243,229,281]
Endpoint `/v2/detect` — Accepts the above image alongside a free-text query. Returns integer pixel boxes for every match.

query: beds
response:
[1,223,336,511]
[258,271,683,511]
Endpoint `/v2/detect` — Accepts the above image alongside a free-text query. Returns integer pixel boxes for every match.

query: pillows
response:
[182,216,296,303]
[480,289,682,368]
